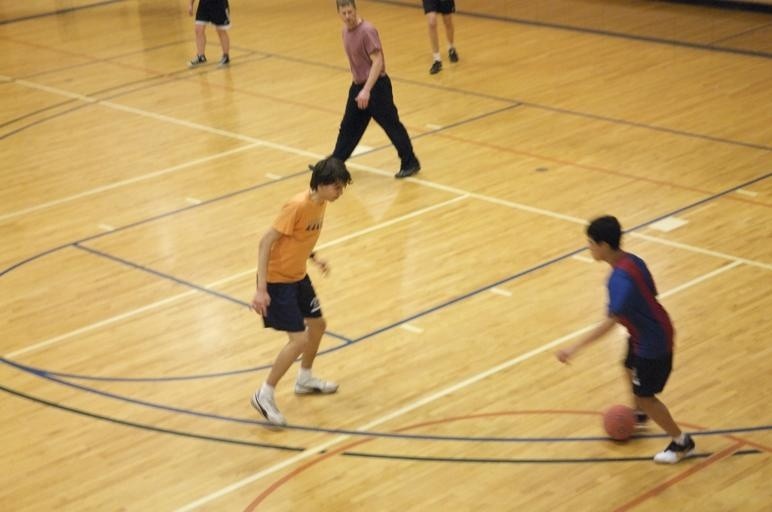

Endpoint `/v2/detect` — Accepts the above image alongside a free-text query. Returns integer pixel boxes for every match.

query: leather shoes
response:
[394,163,421,179]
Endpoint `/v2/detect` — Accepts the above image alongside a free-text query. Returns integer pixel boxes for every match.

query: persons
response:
[309,1,421,180]
[422,0,459,75]
[252,157,351,429]
[188,0,232,69]
[556,215,696,465]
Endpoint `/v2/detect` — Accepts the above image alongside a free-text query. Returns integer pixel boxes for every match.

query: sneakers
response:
[632,407,650,427]
[447,47,459,63]
[186,54,208,68]
[429,60,443,75]
[653,432,696,465]
[294,377,339,395]
[249,388,287,427]
[217,53,231,66]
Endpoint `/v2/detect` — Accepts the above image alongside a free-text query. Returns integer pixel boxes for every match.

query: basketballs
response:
[604,405,636,440]
